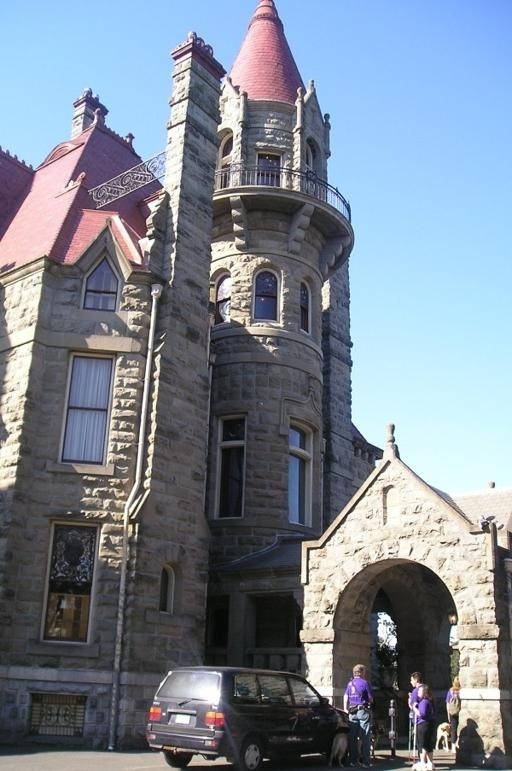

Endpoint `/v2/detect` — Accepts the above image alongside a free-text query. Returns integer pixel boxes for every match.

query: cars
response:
[146,666,348,770]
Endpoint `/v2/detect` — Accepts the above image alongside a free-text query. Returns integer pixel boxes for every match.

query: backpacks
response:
[448,695,460,715]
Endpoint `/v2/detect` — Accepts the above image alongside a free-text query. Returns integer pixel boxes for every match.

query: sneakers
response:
[412,761,435,771]
[350,761,374,768]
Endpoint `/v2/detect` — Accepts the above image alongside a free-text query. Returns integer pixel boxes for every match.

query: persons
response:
[411,684,437,771]
[342,664,375,768]
[445,674,462,752]
[407,671,433,763]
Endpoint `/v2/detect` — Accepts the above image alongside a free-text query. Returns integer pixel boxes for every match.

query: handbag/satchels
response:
[370,703,379,719]
